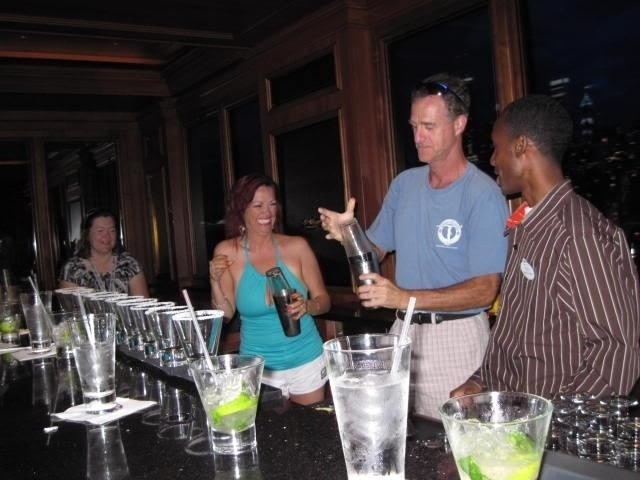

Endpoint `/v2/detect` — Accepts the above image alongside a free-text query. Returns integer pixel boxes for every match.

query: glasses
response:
[414,81,464,103]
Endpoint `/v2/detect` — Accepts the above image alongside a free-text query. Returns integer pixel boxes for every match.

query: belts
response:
[397,310,481,324]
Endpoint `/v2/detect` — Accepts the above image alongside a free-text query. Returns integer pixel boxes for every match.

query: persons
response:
[56,207,152,299]
[318,72,511,421]
[449,91,640,402]
[208,172,332,406]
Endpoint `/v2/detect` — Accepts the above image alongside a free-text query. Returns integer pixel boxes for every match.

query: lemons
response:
[459,430,541,480]
[1,322,16,332]
[214,390,256,416]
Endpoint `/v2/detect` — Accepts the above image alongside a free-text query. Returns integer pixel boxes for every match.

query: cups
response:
[341,217,383,311]
[16,290,53,352]
[440,389,553,479]
[189,354,262,455]
[265,267,302,337]
[63,313,121,414]
[57,284,226,373]
[320,332,414,480]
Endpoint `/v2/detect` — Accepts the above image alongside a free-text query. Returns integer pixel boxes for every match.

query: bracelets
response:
[213,296,229,309]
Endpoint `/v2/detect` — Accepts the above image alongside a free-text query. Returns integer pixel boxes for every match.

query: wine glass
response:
[541,392,640,471]
